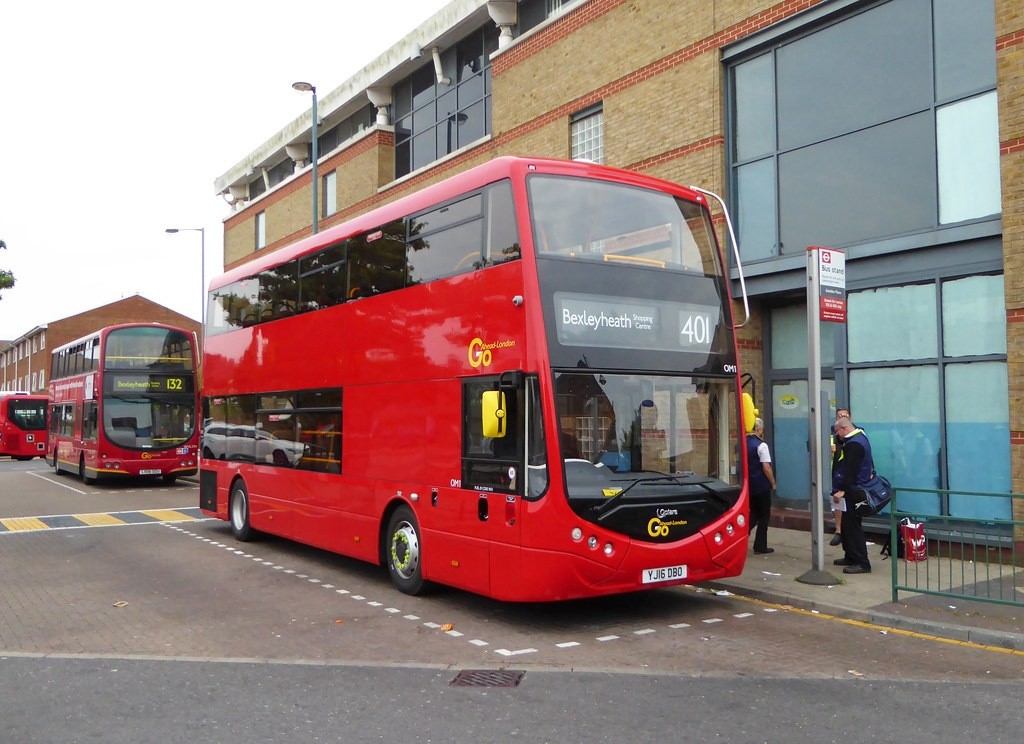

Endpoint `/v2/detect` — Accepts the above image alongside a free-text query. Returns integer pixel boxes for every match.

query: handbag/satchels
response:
[851,476,892,516]
[900,516,928,563]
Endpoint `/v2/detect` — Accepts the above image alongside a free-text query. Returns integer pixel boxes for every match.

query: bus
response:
[193,153,758,603]
[47,322,199,487]
[0,390,51,461]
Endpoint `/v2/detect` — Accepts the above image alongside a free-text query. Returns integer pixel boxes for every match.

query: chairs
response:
[228,286,388,333]
[103,359,184,369]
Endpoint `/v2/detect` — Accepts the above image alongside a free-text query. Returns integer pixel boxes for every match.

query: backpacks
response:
[882,522,905,558]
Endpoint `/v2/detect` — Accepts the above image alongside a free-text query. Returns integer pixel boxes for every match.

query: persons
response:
[733,415,779,556]
[833,415,876,575]
[828,407,867,547]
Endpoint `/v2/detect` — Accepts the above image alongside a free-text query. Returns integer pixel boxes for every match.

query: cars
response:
[202,420,311,468]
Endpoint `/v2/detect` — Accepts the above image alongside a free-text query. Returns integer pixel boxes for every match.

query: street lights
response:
[165,226,205,363]
[292,81,319,234]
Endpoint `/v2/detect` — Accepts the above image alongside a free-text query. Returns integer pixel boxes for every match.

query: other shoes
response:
[755,547,774,554]
[834,558,855,565]
[830,532,842,546]
[844,565,871,574]
